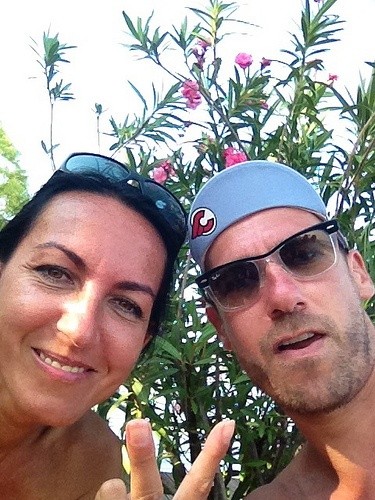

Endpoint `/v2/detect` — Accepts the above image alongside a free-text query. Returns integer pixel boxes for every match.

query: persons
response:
[187,159,375,500]
[1,151,236,499]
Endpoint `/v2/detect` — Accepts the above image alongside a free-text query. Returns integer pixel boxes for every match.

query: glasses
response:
[47,153,187,263]
[195,219,349,313]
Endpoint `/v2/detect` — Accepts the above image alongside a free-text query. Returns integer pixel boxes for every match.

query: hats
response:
[188,160,329,274]
